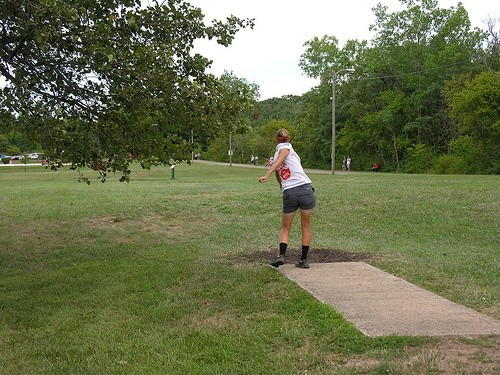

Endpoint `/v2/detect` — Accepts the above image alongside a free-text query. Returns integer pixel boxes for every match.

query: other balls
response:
[253,111,259,118]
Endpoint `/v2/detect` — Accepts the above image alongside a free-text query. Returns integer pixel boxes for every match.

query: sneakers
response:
[295,259,309,268]
[270,254,285,267]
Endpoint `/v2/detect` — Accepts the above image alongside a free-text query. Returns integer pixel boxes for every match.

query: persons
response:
[40,158,45,166]
[195,152,202,160]
[346,156,351,171]
[265,156,274,167]
[251,155,258,166]
[372,161,379,172]
[342,155,346,170]
[258,128,316,268]
